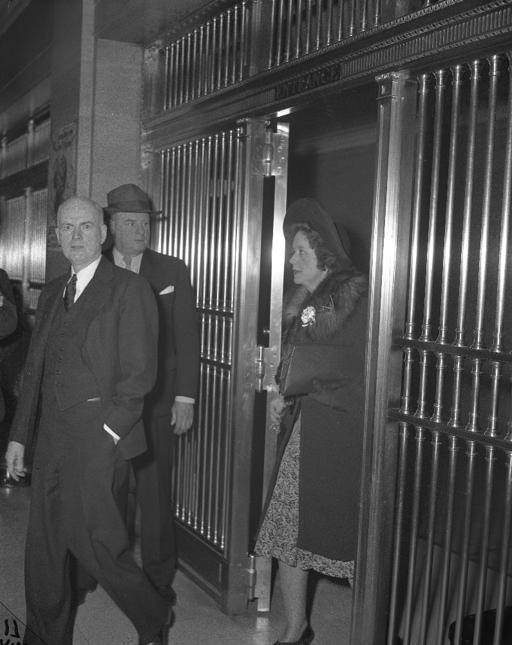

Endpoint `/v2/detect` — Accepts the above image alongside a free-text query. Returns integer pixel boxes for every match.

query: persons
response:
[1,196,177,645]
[250,194,371,645]
[0,269,30,486]
[68,184,202,627]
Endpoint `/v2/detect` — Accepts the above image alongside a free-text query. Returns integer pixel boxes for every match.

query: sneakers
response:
[152,583,179,606]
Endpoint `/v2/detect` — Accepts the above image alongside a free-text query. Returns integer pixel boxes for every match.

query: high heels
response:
[271,622,317,645]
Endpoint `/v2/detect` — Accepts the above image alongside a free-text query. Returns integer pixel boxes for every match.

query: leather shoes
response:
[136,600,174,645]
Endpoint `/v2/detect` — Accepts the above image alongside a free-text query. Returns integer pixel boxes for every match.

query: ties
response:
[61,273,77,313]
[122,255,134,273]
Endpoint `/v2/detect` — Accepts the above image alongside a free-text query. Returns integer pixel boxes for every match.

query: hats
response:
[282,194,358,274]
[100,183,164,217]
